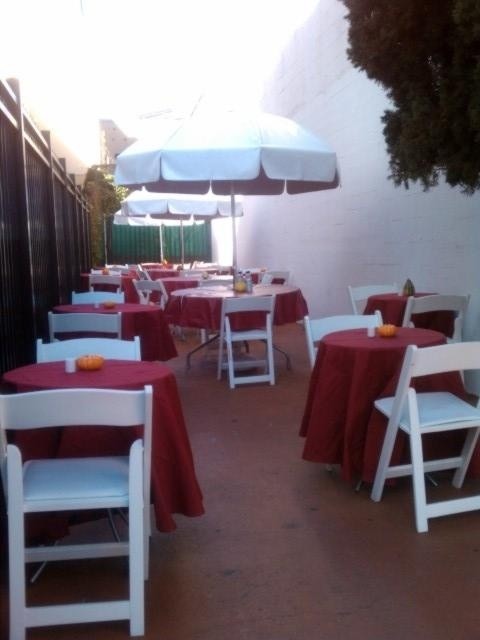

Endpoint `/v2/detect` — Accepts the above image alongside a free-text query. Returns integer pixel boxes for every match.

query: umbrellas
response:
[112,209,205,262]
[119,185,243,262]
[112,106,343,284]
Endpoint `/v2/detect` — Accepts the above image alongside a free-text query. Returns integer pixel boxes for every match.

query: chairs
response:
[402,293,471,388]
[36,261,307,390]
[370,341,480,535]
[348,283,385,317]
[0,384,153,640]
[303,310,384,372]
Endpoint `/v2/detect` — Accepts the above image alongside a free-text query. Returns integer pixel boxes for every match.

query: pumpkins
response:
[377,324,397,338]
[76,355,104,371]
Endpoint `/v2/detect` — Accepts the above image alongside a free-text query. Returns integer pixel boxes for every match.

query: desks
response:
[362,292,455,339]
[0,358,206,586]
[298,326,480,492]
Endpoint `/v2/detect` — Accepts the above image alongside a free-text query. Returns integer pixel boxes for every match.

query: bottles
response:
[237,271,243,279]
[245,270,253,293]
